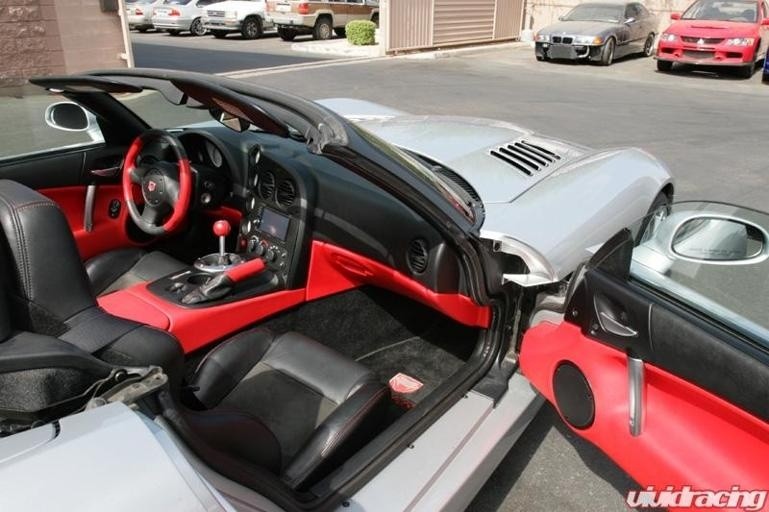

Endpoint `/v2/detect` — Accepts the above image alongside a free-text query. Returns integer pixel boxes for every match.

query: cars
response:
[535,0,653,64]
[653,0,769,77]
[125,0,379,43]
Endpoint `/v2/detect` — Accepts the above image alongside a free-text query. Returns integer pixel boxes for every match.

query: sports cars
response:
[0,46,769,511]
